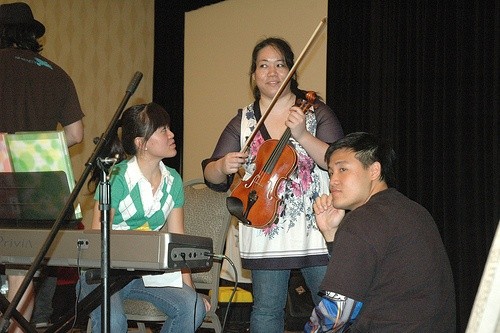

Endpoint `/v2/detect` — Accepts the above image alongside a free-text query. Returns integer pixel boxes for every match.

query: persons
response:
[75,103,212,333]
[303,132,456,333]
[0,2,86,333]
[202,39,346,333]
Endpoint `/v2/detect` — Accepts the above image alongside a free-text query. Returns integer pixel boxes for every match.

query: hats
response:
[0,2,45,38]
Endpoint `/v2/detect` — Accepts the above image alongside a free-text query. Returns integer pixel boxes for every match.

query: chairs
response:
[86,179,231,333]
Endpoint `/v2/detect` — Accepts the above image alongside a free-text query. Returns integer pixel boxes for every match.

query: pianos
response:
[0,229,214,272]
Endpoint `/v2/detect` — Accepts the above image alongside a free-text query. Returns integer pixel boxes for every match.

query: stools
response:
[208,287,254,333]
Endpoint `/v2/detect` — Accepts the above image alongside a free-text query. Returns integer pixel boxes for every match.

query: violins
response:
[226,91,319,229]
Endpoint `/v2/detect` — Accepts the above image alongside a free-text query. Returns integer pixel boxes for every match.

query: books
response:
[0,132,21,219]
[5,130,83,220]
